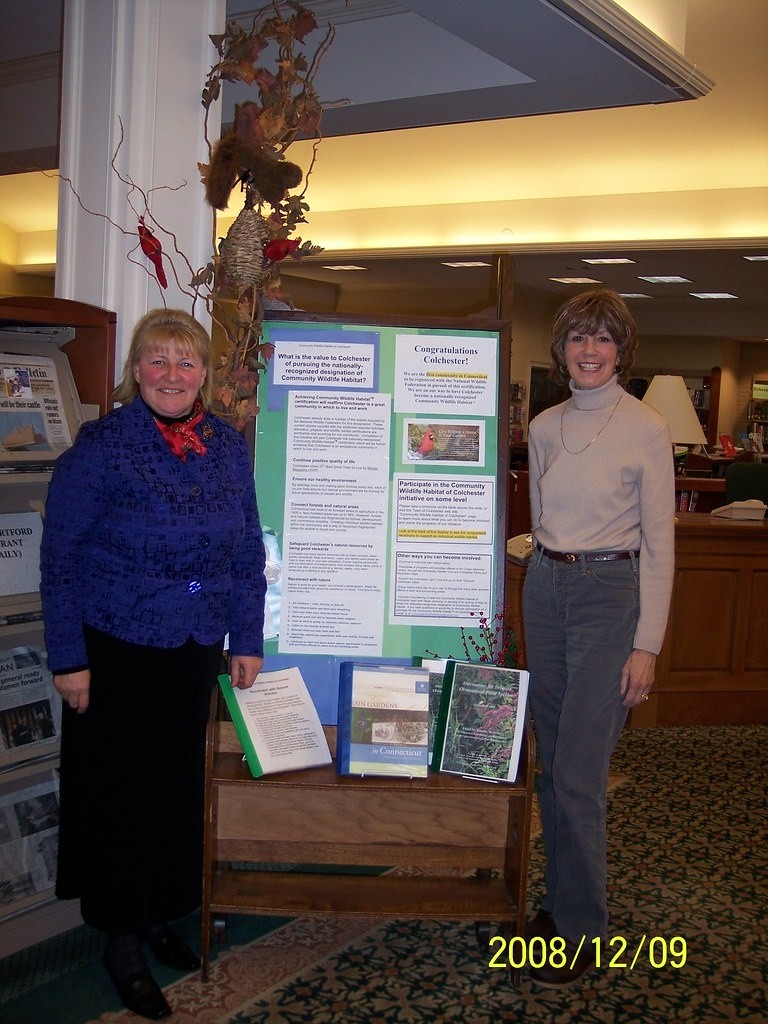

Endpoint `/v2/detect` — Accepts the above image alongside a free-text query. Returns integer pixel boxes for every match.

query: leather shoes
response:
[150,930,200,972]
[101,940,173,1020]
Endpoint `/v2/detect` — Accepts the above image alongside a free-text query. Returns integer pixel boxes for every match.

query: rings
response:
[641,694,649,701]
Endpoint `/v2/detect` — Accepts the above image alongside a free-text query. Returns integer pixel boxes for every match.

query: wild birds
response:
[137,215,168,289]
[262,236,302,261]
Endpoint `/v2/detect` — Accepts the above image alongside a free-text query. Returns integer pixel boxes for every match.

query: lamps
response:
[642,373,708,458]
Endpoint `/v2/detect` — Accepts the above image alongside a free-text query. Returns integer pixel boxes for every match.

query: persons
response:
[39,307,267,1019]
[523,289,675,991]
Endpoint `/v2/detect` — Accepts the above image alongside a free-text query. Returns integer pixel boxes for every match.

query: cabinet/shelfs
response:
[199,686,537,990]
[0,294,119,959]
[684,366,722,454]
[745,375,768,453]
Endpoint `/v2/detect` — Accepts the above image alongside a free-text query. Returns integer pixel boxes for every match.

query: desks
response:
[698,452,735,478]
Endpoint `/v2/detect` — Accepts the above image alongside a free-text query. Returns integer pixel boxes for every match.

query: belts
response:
[536,540,640,564]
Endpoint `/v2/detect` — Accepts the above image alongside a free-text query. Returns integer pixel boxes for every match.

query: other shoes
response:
[529,941,603,990]
[523,907,558,957]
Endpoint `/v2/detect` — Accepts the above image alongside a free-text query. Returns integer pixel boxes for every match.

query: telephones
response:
[507,532,534,566]
[711,499,768,520]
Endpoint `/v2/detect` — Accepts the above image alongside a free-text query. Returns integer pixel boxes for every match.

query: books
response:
[753,380,768,399]
[509,383,524,446]
[217,661,530,786]
[718,400,768,458]
[680,490,698,513]
[688,389,705,407]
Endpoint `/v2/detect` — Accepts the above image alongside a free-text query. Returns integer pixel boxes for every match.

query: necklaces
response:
[561,397,623,455]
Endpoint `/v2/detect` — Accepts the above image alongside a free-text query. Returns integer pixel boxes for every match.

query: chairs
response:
[749,440,768,463]
